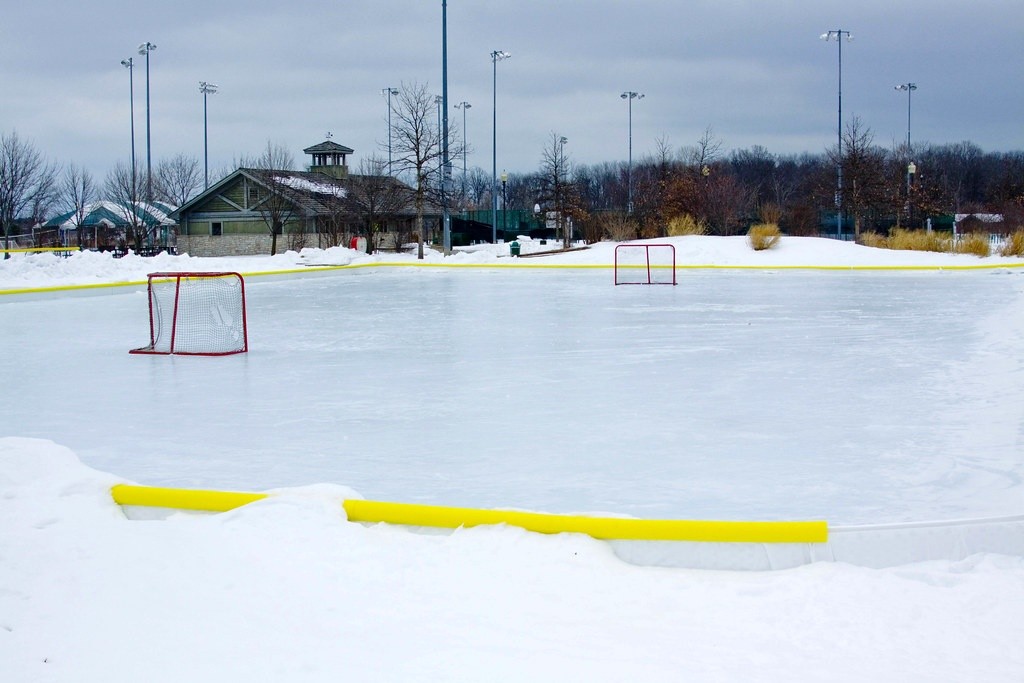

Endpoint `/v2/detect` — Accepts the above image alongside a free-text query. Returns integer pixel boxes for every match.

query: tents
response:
[34,201,177,248]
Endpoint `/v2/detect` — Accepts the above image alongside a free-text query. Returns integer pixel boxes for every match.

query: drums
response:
[348,235,367,253]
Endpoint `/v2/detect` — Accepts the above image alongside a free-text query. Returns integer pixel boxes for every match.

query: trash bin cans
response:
[509,242,521,256]
[432,236,438,244]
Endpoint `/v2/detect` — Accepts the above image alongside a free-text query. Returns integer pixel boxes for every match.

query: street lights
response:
[119,57,139,257]
[906,161,918,234]
[137,40,158,206]
[559,135,568,181]
[455,100,473,189]
[434,94,444,191]
[198,80,219,190]
[819,28,854,241]
[621,89,648,218]
[892,82,916,191]
[501,168,511,243]
[381,86,400,180]
[489,49,511,244]
